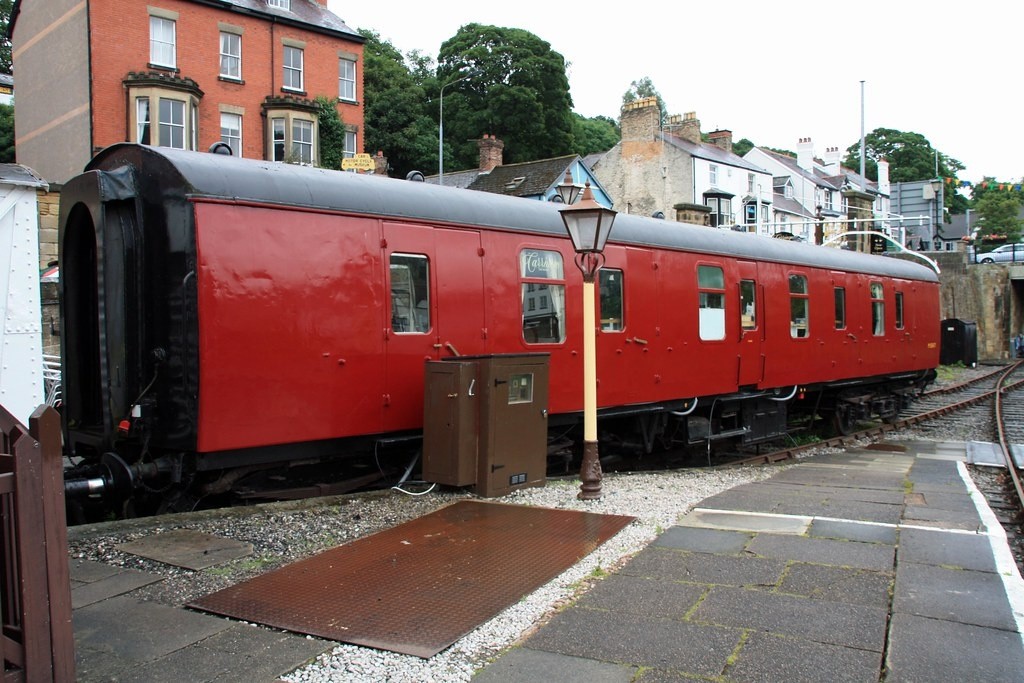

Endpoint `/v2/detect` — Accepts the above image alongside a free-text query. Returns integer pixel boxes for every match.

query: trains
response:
[0,142,941,526]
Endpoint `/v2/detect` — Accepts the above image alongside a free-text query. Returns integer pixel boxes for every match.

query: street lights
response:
[931,176,942,251]
[558,177,618,499]
[438,71,484,185]
[802,162,836,232]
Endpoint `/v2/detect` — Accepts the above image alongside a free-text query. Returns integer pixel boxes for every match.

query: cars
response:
[976,243,1024,264]
